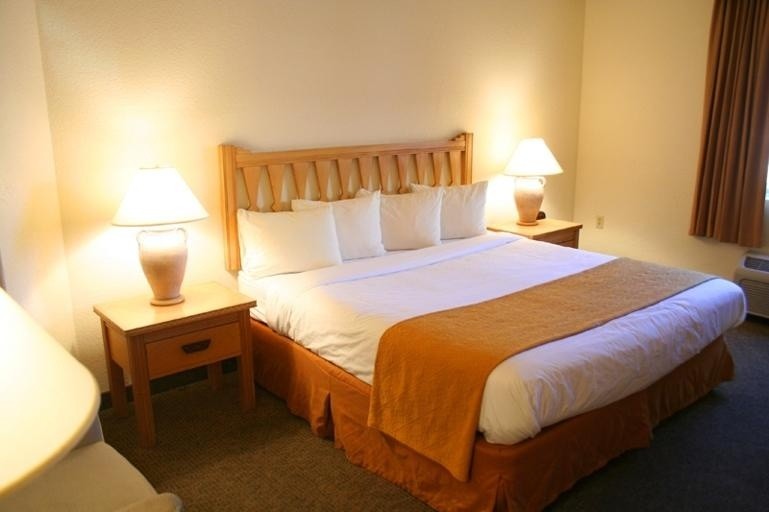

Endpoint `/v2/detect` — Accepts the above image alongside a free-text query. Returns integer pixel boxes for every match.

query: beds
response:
[212,130,746,510]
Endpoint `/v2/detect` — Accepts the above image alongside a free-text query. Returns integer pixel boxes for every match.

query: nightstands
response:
[487,217,583,250]
[92,281,258,450]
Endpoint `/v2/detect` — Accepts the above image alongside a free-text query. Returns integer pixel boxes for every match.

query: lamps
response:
[109,166,209,308]
[505,136,564,228]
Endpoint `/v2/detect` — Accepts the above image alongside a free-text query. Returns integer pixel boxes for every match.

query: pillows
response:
[235,181,490,284]
